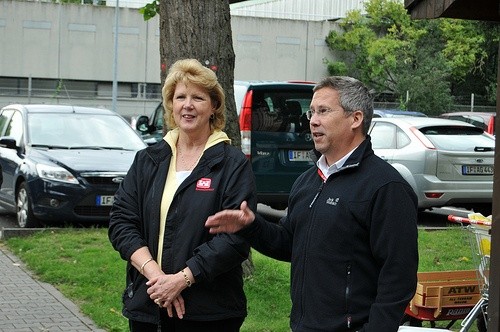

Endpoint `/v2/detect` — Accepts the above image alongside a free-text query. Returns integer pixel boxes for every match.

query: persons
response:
[205,76,419,332]
[108,58,258,332]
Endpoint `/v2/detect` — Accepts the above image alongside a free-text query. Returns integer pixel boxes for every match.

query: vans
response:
[137,80,323,209]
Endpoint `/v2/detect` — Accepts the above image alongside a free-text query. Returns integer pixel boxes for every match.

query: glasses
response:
[306,108,353,120]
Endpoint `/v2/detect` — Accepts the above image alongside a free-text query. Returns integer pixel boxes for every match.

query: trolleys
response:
[447,215,493,332]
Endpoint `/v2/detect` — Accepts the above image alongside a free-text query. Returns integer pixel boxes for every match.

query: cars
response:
[0,105,152,229]
[366,109,495,216]
[445,112,495,136]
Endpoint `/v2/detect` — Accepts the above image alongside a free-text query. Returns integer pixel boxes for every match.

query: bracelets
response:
[139,258,153,273]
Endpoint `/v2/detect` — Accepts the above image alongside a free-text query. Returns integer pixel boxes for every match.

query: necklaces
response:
[178,145,203,171]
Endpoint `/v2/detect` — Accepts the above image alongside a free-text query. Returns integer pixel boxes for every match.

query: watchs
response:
[182,270,192,287]
